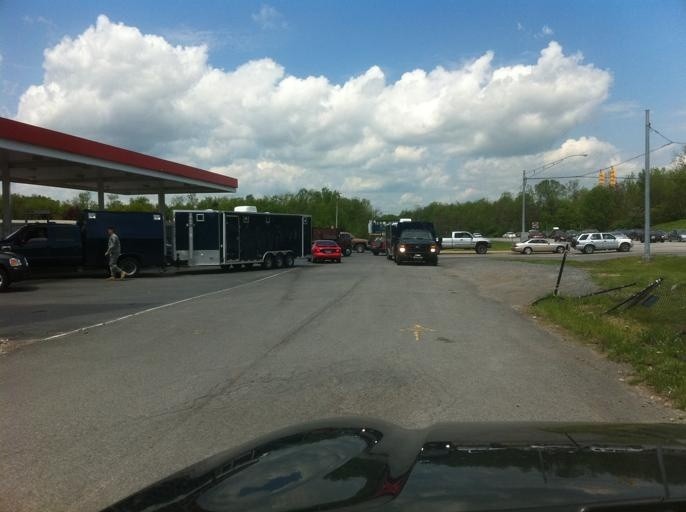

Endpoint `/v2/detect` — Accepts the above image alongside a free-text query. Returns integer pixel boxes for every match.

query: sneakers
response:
[120,271,126,280]
[107,277,116,281]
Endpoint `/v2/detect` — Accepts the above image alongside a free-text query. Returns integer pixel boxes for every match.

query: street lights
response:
[520,153,587,242]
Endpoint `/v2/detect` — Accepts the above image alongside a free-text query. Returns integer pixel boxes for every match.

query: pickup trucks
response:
[436,230,491,254]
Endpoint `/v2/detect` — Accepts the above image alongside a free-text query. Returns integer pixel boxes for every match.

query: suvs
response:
[338,231,368,253]
[572,232,631,254]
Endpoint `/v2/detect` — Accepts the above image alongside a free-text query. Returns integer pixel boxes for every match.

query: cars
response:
[502,229,600,246]
[310,240,341,263]
[100,417,685,512]
[511,238,569,255]
[620,228,686,243]
[0,250,30,291]
[472,232,483,237]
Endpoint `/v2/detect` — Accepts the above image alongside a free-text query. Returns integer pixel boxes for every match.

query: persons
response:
[104,226,127,280]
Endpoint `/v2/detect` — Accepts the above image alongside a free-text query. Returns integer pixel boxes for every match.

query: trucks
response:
[311,228,351,256]
[0,211,166,278]
[384,221,438,265]
[367,215,412,255]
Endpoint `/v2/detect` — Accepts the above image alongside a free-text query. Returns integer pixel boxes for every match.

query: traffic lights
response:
[597,169,605,185]
[608,168,616,187]
